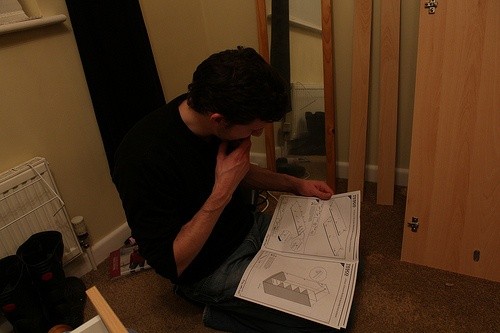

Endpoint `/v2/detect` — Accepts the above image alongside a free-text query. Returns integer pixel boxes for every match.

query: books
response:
[109,246,151,280]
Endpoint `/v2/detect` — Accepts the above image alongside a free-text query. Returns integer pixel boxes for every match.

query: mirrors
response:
[256,0,336,193]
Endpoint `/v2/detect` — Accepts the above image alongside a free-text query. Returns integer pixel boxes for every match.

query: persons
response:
[120,45,336,333]
[129,247,145,270]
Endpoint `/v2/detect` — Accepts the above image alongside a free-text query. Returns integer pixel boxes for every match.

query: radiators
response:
[286,81,324,141]
[0,156,83,266]
